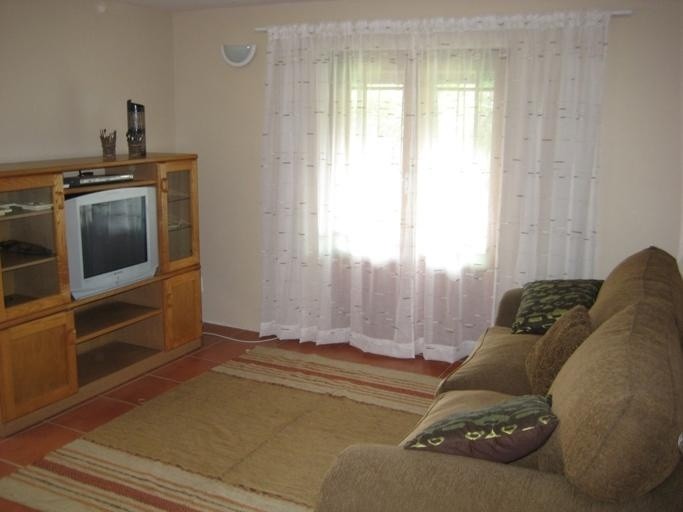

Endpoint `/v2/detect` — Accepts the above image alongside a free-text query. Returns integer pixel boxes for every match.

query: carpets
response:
[0,345,442,512]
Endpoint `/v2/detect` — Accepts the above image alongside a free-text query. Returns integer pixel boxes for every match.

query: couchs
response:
[313,247,683,512]
[313,287,683,512]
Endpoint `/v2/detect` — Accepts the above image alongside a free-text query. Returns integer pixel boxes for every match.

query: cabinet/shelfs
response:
[1,154,203,424]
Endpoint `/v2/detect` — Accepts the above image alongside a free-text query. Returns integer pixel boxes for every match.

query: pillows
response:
[525,305,597,397]
[510,279,605,336]
[404,391,561,463]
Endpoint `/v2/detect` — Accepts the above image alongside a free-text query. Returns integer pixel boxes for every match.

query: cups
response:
[127,100,146,156]
[127,140,142,159]
[102,143,115,162]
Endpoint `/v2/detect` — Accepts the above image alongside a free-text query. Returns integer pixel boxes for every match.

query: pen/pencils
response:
[100,128,116,144]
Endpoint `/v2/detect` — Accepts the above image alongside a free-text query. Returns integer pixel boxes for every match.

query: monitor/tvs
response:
[64,186,159,301]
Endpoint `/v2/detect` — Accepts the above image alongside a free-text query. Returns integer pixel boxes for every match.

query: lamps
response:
[220,44,256,67]
